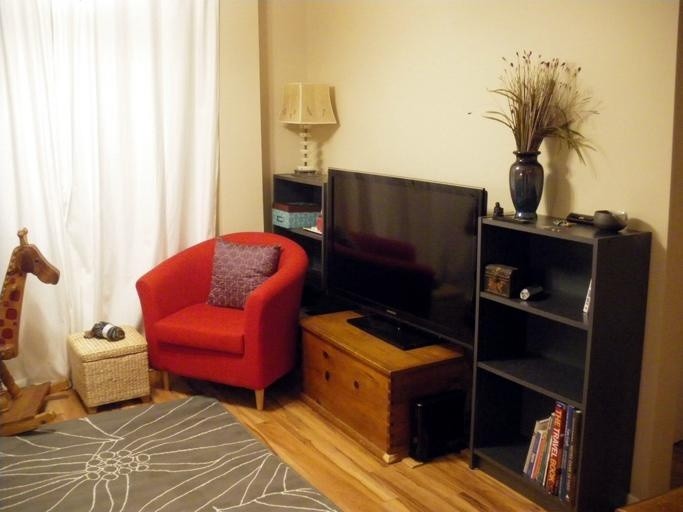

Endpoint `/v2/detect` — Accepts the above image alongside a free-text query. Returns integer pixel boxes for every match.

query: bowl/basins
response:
[594,210,627,232]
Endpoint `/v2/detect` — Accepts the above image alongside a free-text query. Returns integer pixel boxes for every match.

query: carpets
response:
[0,395,341,512]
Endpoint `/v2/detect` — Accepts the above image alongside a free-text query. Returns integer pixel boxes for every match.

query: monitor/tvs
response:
[325,168,487,350]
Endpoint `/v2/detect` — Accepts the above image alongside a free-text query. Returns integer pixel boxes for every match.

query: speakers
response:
[410,388,466,460]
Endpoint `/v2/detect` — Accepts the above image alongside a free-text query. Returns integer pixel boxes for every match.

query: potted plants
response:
[483,50,601,222]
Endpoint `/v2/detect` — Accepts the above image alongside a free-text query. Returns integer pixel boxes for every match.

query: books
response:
[522,401,582,506]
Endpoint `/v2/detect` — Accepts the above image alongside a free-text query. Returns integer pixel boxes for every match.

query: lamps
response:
[279,84,338,173]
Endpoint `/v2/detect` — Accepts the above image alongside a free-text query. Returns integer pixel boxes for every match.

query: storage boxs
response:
[275,201,320,229]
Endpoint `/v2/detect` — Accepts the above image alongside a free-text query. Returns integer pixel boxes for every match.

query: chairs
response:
[136,231,308,411]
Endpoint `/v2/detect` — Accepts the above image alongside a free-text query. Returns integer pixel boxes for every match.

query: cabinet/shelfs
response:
[299,313,458,463]
[272,173,325,310]
[470,213,652,512]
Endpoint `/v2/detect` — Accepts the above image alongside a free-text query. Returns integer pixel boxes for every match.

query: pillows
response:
[208,235,281,311]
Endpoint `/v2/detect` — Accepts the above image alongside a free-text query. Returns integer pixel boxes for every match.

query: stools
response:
[66,325,152,415]
